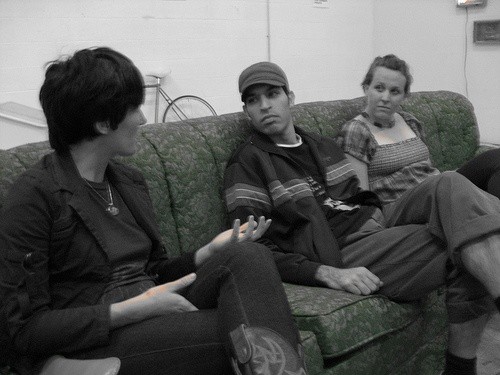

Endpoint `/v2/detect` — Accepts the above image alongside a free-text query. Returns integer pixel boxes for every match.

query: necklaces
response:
[83,173,119,216]
[361,112,396,128]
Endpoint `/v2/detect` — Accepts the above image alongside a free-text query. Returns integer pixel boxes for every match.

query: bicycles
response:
[141,73,219,124]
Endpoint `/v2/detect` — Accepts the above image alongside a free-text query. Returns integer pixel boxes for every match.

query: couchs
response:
[0,91,499,375]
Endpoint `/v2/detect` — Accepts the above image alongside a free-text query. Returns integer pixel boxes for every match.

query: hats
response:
[239,62,288,97]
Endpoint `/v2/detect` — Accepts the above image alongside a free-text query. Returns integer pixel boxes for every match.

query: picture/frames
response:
[473,20,500,43]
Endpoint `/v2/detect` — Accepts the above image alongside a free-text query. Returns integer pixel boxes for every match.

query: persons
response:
[224,62,500,375]
[0,47,304,375]
[336,54,500,204]
